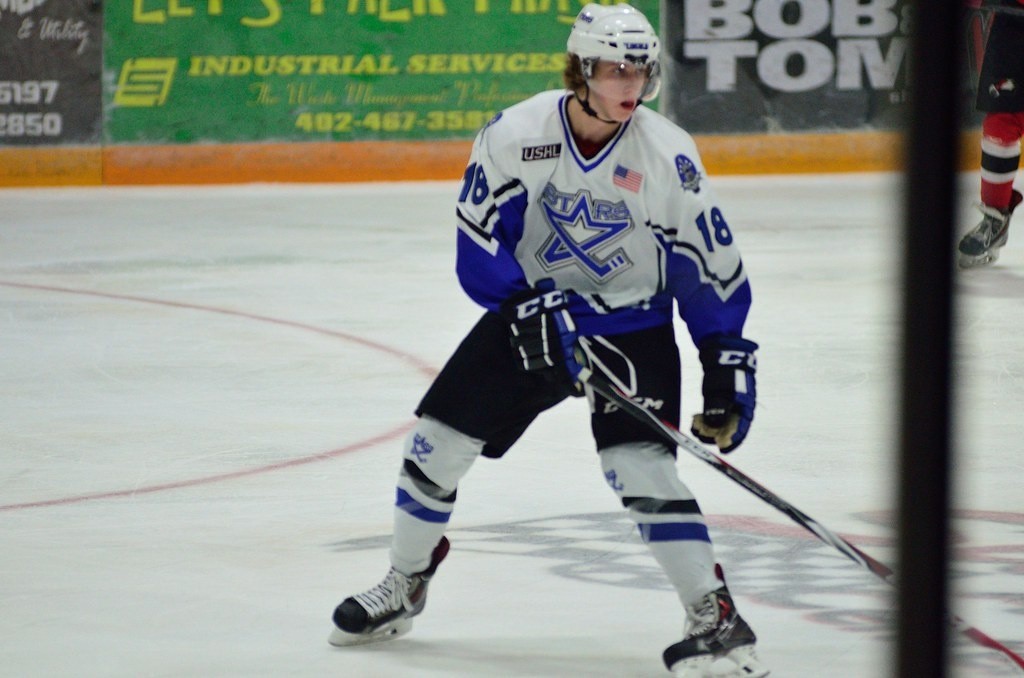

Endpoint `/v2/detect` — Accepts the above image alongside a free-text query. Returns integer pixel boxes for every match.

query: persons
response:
[329,4,770,678]
[956,0,1024,268]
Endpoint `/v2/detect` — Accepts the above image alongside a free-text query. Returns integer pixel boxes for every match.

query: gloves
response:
[691,337,759,453]
[501,289,576,389]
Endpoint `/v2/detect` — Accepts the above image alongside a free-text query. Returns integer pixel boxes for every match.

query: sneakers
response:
[663,564,770,678]
[329,535,450,647]
[958,189,1023,267]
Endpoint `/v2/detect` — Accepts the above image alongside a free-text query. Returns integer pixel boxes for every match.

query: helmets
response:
[567,3,661,79]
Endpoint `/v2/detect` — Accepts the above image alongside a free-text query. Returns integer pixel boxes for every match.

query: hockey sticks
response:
[571,366,1024,676]
